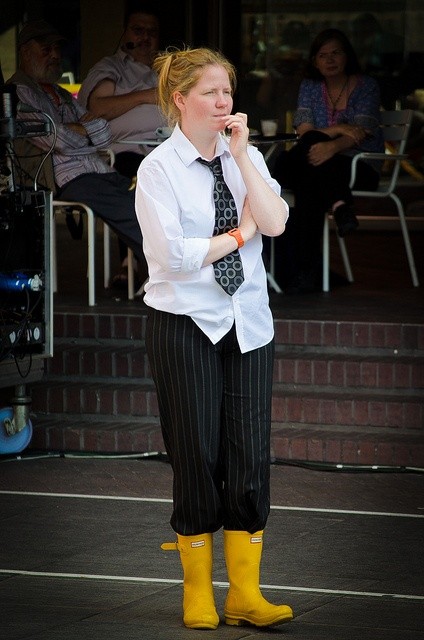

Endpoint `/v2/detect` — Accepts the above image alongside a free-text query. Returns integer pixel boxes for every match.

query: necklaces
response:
[325,78,348,116]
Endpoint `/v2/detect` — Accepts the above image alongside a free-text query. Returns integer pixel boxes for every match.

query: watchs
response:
[227,228,244,249]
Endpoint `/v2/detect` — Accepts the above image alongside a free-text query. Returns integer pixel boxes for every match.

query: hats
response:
[17,20,70,46]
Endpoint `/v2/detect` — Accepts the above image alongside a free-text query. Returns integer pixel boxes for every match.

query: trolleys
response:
[0,81,62,458]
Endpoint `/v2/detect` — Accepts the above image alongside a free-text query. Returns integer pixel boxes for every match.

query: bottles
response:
[260,120,278,138]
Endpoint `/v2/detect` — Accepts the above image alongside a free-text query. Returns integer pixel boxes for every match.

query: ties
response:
[198,158,244,296]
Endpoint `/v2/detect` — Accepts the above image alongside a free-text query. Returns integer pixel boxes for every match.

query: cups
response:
[155,126,173,138]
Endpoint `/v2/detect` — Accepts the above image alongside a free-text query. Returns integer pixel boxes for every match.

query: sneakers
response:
[333,203,360,237]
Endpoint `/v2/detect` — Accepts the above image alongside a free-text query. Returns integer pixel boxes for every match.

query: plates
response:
[247,128,259,138]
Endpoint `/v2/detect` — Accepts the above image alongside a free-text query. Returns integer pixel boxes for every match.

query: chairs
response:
[12,117,109,305]
[320,109,419,292]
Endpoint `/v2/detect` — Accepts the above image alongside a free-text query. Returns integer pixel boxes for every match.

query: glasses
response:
[129,24,159,37]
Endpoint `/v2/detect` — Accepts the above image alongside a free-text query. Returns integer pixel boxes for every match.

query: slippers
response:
[110,266,139,293]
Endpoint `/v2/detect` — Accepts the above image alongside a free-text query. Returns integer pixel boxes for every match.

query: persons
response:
[5,20,148,279]
[77,1,172,296]
[134,46,294,630]
[281,28,385,292]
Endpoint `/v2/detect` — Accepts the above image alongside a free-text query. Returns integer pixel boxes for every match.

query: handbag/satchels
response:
[109,151,144,178]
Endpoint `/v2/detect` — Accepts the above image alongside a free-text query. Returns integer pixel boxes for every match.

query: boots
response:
[161,533,220,629]
[223,530,293,627]
